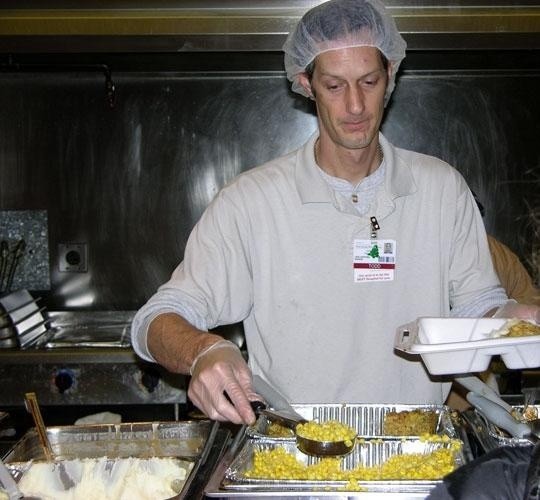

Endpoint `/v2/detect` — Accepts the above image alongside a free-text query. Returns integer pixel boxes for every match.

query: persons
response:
[445,190,540,414]
[130,0,540,427]
